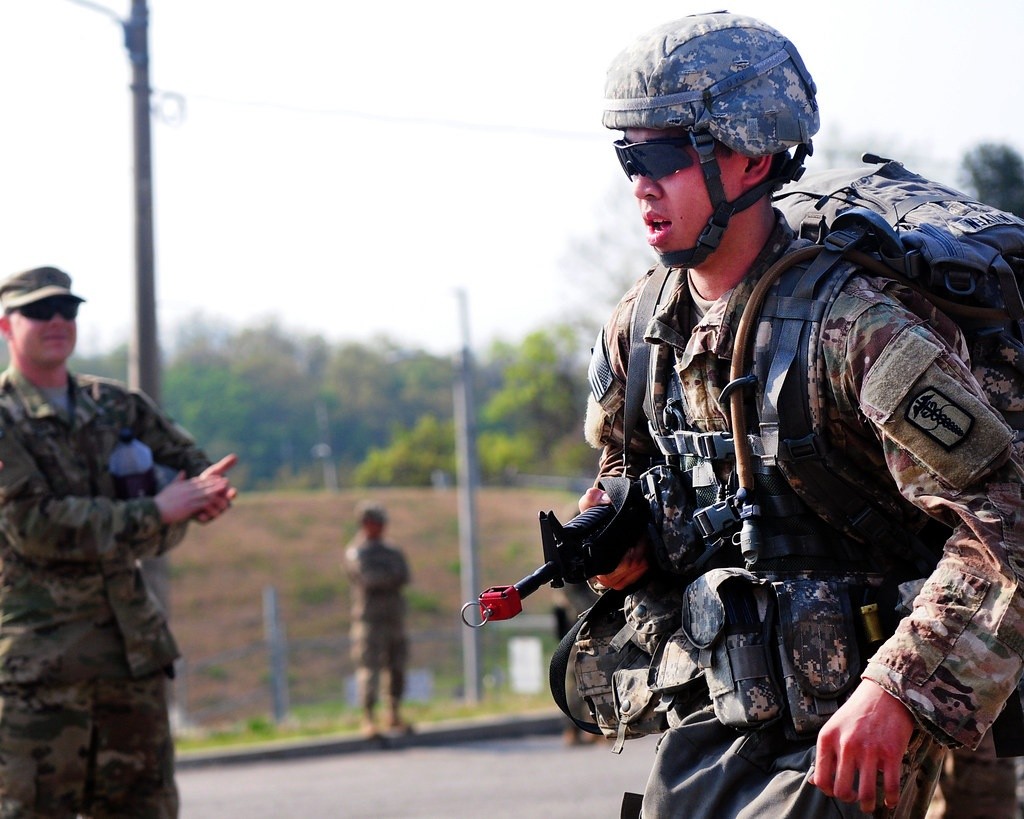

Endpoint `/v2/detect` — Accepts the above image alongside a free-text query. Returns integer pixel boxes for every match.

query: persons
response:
[341,500,414,737]
[0,268,240,819]
[574,9,1023,819]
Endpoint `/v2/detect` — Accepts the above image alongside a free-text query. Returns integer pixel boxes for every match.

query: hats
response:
[357,505,390,523]
[0,267,86,312]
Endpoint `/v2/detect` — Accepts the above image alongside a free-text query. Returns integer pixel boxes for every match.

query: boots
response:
[365,696,411,741]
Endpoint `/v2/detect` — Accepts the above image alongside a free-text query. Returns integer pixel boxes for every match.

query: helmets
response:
[603,11,820,156]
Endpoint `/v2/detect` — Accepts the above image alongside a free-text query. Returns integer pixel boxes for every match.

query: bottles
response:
[108,428,161,559]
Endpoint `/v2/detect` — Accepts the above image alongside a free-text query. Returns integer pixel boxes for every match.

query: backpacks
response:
[628,151,1024,555]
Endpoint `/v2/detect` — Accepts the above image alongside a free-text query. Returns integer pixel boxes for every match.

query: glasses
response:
[611,137,695,182]
[20,300,78,322]
